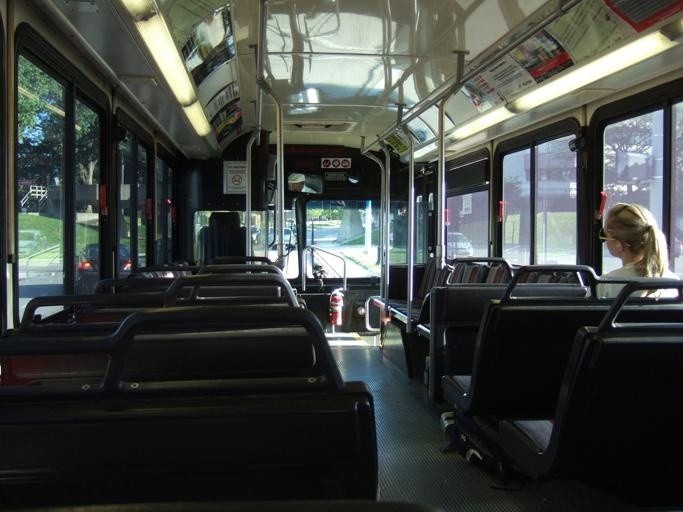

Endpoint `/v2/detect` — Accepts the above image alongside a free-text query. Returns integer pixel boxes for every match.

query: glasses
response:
[598,227,632,248]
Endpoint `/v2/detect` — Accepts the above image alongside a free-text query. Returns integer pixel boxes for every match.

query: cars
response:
[447,231,473,259]
[251,220,296,249]
[19,230,47,257]
[76,243,142,288]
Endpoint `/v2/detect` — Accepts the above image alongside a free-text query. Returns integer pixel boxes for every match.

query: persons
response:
[579,202,682,299]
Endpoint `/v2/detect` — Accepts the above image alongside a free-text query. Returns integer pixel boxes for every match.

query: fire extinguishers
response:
[329,287,344,326]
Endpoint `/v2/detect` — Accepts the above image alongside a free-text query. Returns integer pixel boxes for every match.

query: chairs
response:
[364,212,683,511]
[3,212,383,509]
